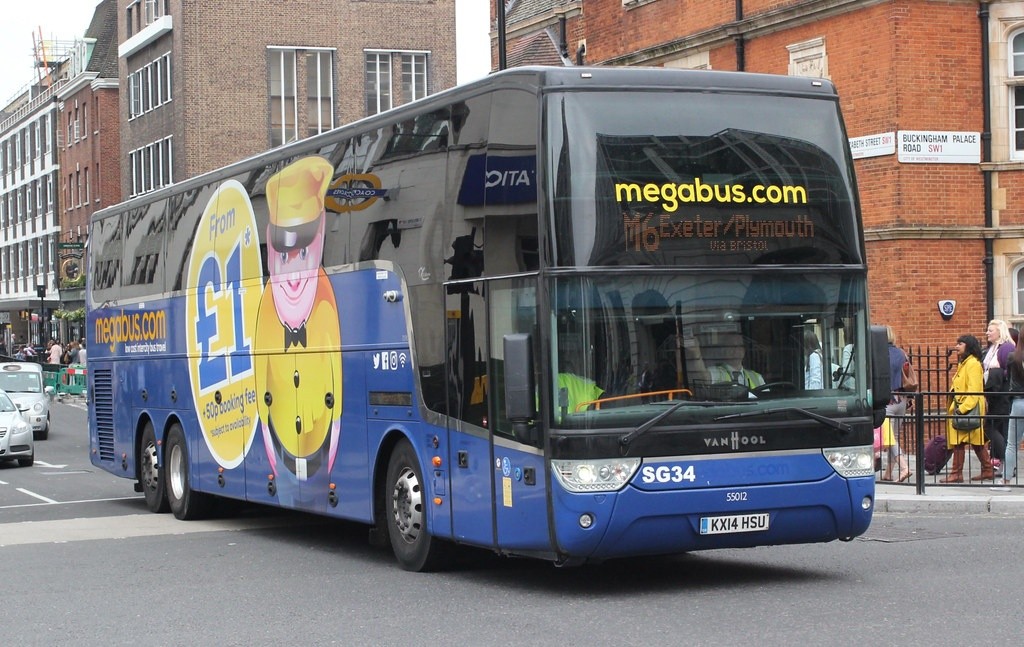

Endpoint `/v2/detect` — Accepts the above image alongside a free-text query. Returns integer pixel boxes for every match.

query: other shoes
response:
[990,482,1012,492]
[874,455,881,471]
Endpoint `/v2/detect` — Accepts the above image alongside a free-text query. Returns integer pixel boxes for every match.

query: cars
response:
[0,388,35,467]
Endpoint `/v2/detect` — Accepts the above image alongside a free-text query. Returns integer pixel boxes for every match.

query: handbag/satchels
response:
[874,428,883,452]
[882,417,895,447]
[63,352,72,363]
[952,401,980,430]
[901,354,919,393]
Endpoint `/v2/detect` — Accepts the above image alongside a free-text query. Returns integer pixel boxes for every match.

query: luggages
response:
[924,436,954,475]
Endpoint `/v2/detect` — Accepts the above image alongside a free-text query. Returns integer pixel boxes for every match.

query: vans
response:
[0,363,56,441]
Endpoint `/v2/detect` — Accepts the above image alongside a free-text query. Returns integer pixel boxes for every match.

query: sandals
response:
[881,473,893,481]
[897,471,913,482]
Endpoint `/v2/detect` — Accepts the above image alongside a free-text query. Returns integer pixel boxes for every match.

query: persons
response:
[535,339,604,422]
[13,344,38,360]
[0,338,9,356]
[607,356,664,404]
[11,334,26,354]
[45,340,86,399]
[803,326,913,483]
[676,330,770,400]
[940,320,1024,491]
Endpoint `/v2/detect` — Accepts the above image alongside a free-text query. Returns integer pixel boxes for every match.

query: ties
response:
[733,371,742,383]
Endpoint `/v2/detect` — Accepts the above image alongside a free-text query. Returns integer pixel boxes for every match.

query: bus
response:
[85,62,893,571]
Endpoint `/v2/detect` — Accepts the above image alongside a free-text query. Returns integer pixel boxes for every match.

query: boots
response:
[939,451,965,483]
[972,449,993,481]
